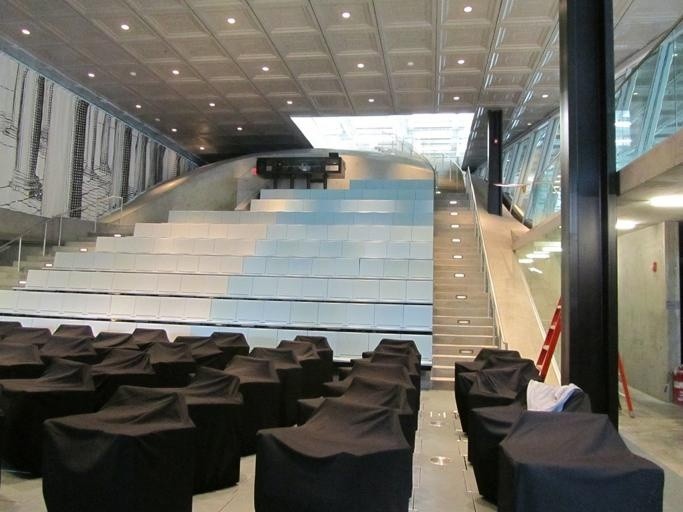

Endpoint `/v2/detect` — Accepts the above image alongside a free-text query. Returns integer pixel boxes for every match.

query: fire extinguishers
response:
[673,366,683,406]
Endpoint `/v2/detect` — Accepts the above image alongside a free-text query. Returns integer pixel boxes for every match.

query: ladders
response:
[536,296,634,416]
